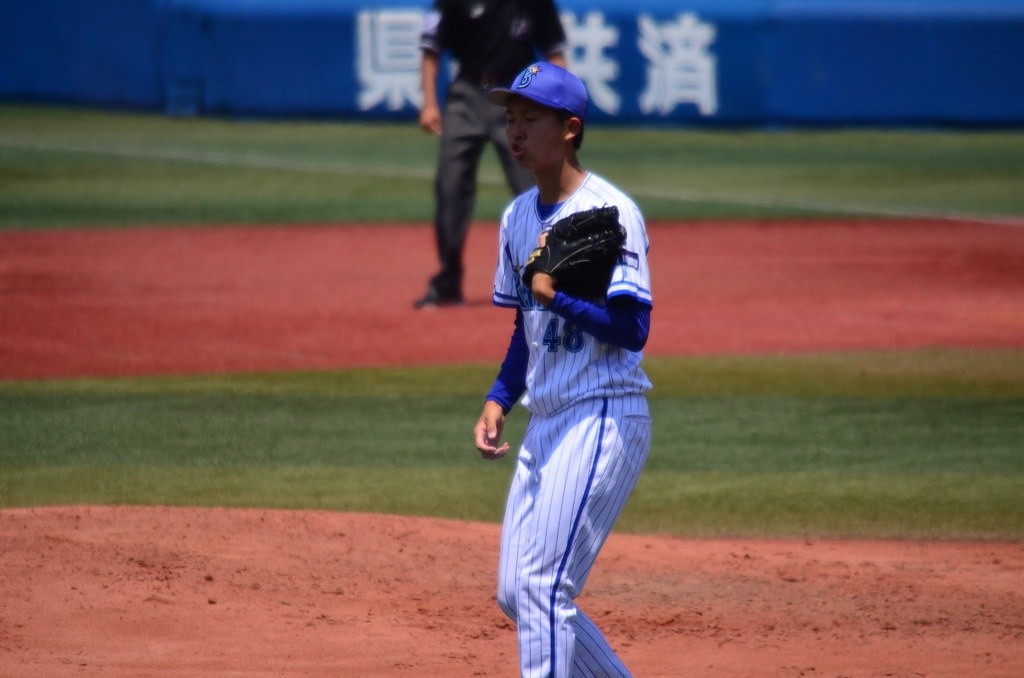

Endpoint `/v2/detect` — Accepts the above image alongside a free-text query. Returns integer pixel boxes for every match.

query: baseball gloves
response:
[520,204,628,295]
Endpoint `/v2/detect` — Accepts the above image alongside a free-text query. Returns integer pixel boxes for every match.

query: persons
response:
[473,62,653,678]
[412,1,567,308]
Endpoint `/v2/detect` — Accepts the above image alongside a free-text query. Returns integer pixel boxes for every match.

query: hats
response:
[486,59,590,121]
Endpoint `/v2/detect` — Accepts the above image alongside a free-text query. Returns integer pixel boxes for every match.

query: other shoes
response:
[414,294,466,306]
